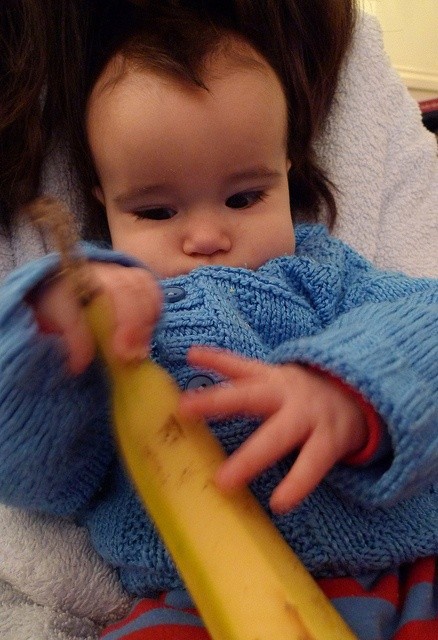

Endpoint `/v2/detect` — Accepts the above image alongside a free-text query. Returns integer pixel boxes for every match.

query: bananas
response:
[34,194,360,640]
